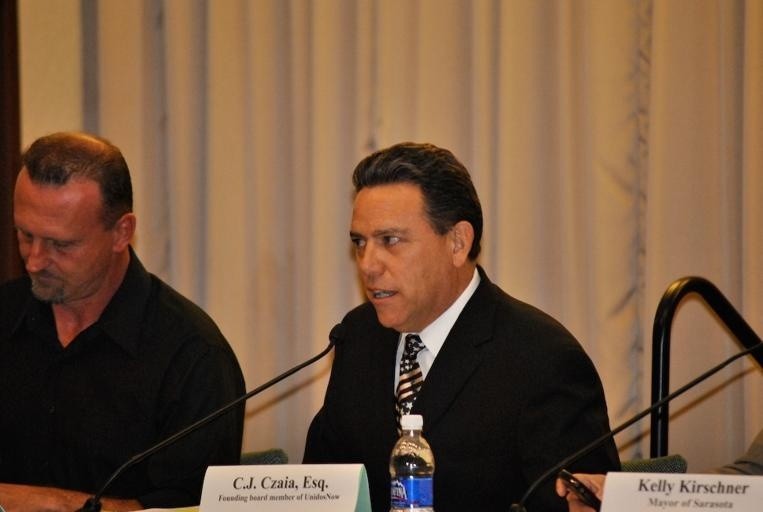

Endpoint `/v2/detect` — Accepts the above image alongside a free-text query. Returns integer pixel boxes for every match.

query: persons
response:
[556,432,763,510]
[0,132,246,511]
[303,143,624,511]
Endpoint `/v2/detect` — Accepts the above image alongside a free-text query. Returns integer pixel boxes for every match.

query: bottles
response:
[389,413,438,512]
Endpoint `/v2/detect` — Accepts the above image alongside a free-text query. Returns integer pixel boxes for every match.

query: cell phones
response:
[558,465,601,512]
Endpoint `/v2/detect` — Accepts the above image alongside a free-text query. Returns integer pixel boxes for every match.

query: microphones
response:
[77,319,346,512]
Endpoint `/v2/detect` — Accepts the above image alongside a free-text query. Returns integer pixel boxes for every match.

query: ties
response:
[395,333,425,441]
